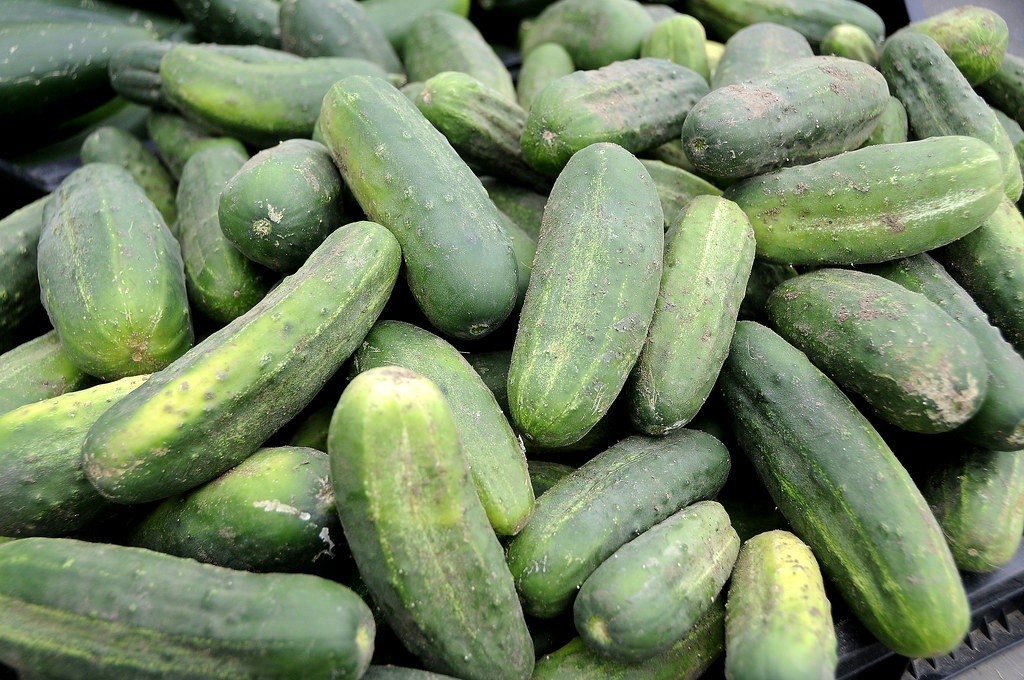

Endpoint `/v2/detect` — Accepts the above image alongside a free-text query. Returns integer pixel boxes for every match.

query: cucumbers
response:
[0,0,1024,680]
